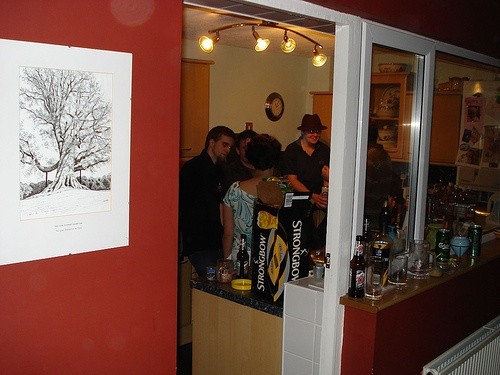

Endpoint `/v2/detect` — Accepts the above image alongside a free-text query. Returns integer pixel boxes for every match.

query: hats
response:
[296,114,327,133]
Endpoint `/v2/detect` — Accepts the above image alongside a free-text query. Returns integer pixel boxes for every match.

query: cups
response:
[206,265,215,280]
[388,253,409,285]
[364,257,386,301]
[215,259,233,283]
[408,239,431,279]
[313,265,325,282]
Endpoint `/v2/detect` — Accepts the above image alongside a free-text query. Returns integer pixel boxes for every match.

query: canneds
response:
[436,229,452,263]
[321,186,328,199]
[371,240,389,286]
[468,224,483,256]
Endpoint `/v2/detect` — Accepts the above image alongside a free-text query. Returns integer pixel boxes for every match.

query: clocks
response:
[265,92,284,122]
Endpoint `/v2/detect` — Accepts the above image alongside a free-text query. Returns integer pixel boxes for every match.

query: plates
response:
[379,85,402,111]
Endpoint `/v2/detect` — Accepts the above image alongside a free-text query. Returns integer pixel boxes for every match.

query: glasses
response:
[304,130,321,134]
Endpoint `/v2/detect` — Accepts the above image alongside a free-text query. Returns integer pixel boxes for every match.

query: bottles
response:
[347,235,367,298]
[235,233,250,279]
[379,178,472,233]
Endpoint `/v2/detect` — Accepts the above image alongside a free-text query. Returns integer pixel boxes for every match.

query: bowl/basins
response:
[377,62,407,73]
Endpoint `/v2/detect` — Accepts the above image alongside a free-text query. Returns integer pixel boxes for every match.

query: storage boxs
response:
[249,183,315,308]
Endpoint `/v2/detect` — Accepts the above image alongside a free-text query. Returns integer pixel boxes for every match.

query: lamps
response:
[199,19,327,67]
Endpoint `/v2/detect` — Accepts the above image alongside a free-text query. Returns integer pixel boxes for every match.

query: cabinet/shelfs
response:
[371,71,414,159]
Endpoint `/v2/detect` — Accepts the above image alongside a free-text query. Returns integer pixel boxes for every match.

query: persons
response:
[179,114,394,281]
